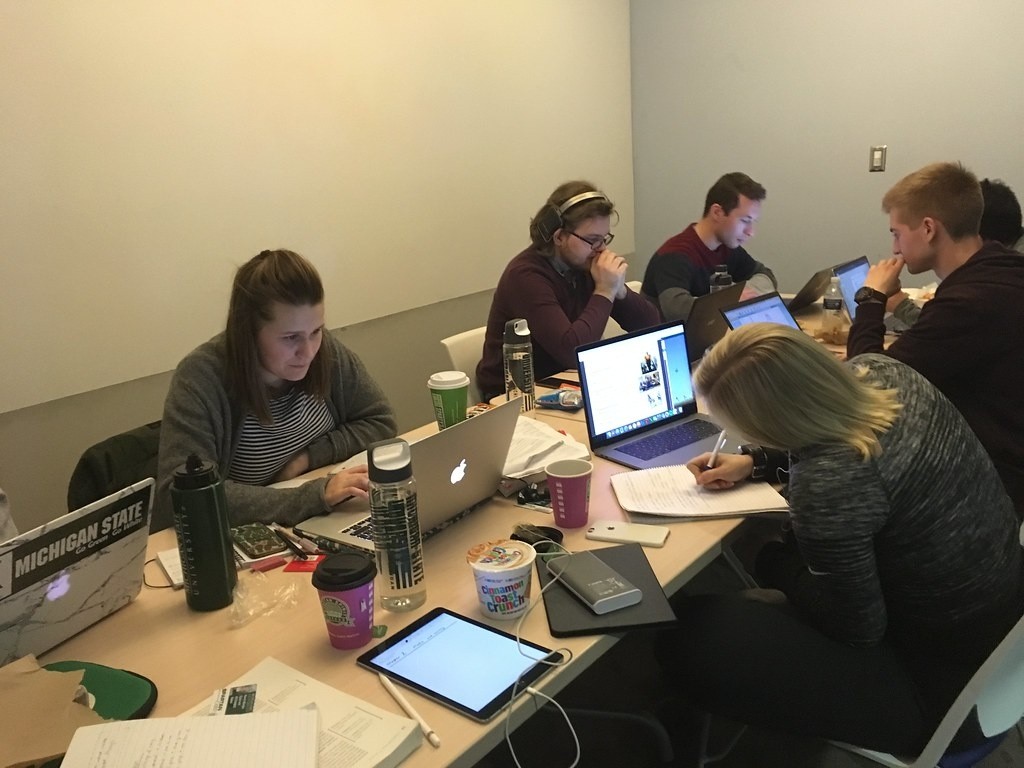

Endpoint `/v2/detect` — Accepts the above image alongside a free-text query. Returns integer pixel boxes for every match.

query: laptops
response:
[683,281,747,363]
[293,394,524,554]
[719,290,803,334]
[0,478,156,669]
[781,262,844,312]
[574,318,750,471]
[831,255,927,336]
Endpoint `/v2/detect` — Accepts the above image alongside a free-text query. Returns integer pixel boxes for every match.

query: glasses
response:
[563,229,615,249]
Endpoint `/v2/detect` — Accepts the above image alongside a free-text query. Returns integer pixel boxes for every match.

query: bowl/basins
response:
[900,287,935,310]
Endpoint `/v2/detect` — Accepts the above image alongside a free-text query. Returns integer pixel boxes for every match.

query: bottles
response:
[502,318,535,419]
[709,265,732,294]
[168,453,238,611]
[367,438,426,613]
[823,277,843,332]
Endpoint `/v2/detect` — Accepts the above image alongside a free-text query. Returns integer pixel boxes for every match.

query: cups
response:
[466,539,536,620]
[312,550,377,649]
[544,459,593,528]
[427,371,470,431]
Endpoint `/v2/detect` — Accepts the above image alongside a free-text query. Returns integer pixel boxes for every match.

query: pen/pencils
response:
[292,528,339,554]
[271,522,320,554]
[698,428,726,492]
[274,529,307,561]
[378,672,440,749]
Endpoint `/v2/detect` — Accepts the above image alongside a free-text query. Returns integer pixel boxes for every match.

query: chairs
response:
[596,280,642,340]
[67,416,162,516]
[440,325,487,409]
[829,614,1023,766]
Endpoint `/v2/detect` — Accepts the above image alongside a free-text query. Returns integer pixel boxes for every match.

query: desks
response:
[0,283,920,767]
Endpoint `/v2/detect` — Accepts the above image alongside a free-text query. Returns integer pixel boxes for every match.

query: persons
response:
[640,174,777,320]
[978,178,1024,255]
[846,161,1024,525]
[650,321,1023,754]
[476,184,660,401]
[150,249,396,536]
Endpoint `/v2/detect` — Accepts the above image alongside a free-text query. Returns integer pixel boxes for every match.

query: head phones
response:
[537,191,608,243]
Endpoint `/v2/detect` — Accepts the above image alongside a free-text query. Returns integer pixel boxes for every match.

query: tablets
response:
[356,606,564,721]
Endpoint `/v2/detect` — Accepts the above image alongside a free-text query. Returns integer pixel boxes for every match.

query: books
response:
[610,463,791,524]
[155,526,303,588]
[62,654,423,768]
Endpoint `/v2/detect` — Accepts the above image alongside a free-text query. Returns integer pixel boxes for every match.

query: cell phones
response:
[231,522,289,559]
[535,378,582,389]
[586,521,671,547]
